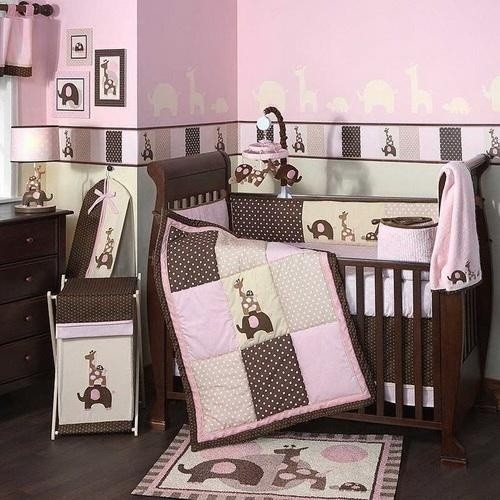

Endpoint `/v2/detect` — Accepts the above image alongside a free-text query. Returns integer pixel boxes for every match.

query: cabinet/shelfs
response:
[0,211,73,402]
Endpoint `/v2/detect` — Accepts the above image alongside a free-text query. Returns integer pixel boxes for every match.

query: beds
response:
[146,150,493,465]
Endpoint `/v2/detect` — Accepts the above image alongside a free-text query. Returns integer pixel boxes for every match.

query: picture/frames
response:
[50,70,90,118]
[64,29,92,67]
[95,49,124,107]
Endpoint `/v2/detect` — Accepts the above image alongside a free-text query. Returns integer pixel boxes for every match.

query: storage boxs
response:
[47,274,143,442]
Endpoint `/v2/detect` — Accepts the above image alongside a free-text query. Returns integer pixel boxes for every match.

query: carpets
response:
[130,423,404,500]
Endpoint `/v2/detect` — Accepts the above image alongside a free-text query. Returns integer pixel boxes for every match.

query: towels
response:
[429,160,483,293]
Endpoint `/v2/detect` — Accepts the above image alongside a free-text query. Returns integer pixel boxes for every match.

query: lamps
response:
[11,125,60,214]
[242,106,293,162]
